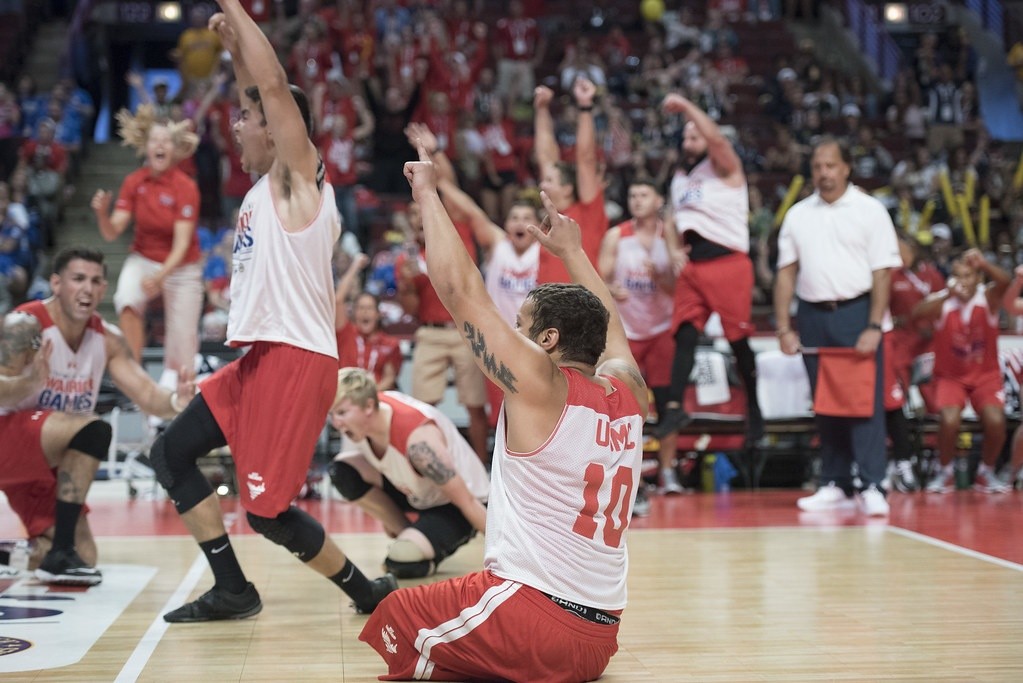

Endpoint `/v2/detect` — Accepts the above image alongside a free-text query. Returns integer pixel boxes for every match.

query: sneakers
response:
[163,581,263,621]
[32,549,102,585]
[928,468,956,494]
[661,477,684,495]
[973,468,1012,495]
[859,483,890,518]
[894,462,920,493]
[371,572,398,607]
[631,489,651,517]
[796,481,859,510]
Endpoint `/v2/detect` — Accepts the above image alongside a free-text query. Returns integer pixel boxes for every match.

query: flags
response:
[816,346,875,416]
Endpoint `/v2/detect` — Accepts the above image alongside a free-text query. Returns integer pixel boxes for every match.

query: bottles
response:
[702,455,717,492]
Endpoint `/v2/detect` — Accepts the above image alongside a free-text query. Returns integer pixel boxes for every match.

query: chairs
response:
[679,341,1018,496]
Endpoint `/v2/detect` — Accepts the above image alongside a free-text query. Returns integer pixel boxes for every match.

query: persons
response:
[357,138,649,683]
[326,366,490,578]
[151,0,398,621]
[0,0,1023,513]
[0,247,198,587]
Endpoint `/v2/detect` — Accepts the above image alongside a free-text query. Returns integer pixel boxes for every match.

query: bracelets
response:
[429,147,440,155]
[170,394,183,414]
[867,324,881,329]
[776,327,791,336]
[576,103,594,110]
